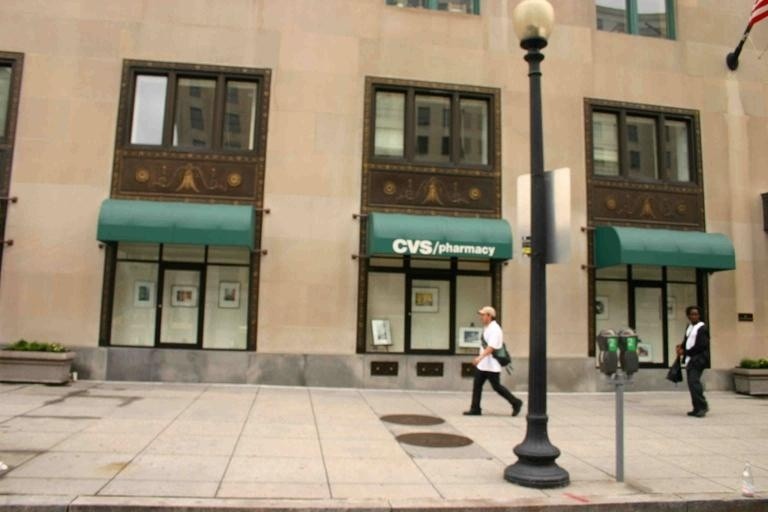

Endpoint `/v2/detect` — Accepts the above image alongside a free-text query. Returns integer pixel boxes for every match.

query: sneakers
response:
[687,407,710,417]
[512,399,523,416]
[464,407,481,415]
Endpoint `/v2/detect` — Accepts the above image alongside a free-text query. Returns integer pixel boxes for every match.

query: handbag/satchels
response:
[666,356,684,384]
[492,345,512,367]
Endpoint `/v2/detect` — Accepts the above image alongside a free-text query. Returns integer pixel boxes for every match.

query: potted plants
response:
[732,358,768,396]
[0,338,75,384]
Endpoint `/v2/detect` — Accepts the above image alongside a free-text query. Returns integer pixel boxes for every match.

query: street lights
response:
[502,1,573,489]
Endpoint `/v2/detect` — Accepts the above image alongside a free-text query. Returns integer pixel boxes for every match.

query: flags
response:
[748,0,768,27]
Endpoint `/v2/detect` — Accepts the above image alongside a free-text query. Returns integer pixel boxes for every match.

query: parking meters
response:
[598,328,640,483]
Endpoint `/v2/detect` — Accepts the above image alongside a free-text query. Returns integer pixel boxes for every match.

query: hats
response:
[478,306,496,317]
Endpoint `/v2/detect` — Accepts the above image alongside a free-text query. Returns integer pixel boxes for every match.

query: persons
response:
[675,305,710,417]
[461,306,524,417]
